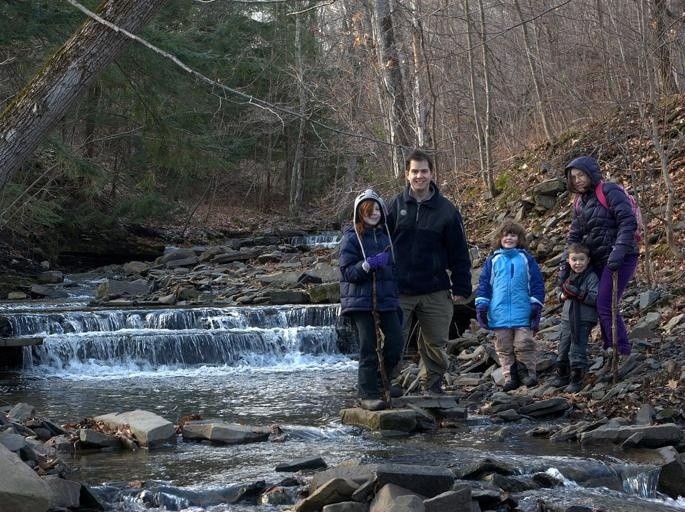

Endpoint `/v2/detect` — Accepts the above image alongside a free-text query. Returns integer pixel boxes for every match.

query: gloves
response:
[560,283,584,302]
[529,303,542,330]
[563,278,578,298]
[558,268,569,286]
[475,303,488,329]
[606,245,626,270]
[367,252,390,269]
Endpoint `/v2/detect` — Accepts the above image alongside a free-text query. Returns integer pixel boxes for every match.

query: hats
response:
[353,189,389,226]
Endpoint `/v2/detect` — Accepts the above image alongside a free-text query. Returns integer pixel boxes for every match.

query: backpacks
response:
[595,180,645,249]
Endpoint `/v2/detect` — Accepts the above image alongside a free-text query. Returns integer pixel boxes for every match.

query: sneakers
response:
[361,396,386,410]
[502,380,520,392]
[390,383,403,398]
[424,389,449,398]
[519,375,538,387]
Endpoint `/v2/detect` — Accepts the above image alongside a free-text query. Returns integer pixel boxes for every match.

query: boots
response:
[589,348,614,374]
[550,367,571,388]
[602,350,638,380]
[563,369,587,394]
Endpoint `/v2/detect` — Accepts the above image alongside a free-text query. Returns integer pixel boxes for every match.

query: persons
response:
[474,219,545,393]
[339,188,404,412]
[554,156,641,382]
[550,242,600,393]
[386,151,473,399]
